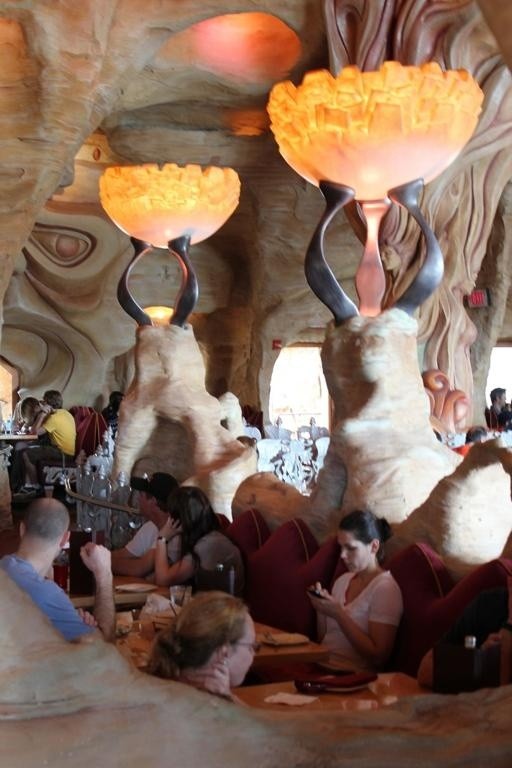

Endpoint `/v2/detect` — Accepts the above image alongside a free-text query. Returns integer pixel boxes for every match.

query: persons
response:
[12,389,76,501]
[489,386,505,413]
[306,507,405,671]
[16,396,52,445]
[142,590,260,707]
[464,426,488,443]
[110,471,182,586]
[101,390,123,417]
[107,394,124,438]
[1,497,119,651]
[152,483,249,595]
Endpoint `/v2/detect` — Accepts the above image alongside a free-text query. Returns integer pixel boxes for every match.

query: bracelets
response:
[157,536,170,545]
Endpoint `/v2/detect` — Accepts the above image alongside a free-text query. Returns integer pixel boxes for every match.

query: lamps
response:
[266,60,487,326]
[95,162,242,328]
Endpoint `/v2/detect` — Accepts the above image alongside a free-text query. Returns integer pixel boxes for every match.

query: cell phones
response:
[308,589,322,599]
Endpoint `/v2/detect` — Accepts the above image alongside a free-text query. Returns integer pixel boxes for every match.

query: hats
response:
[129,471,181,512]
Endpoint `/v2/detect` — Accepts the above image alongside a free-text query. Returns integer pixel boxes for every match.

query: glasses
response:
[226,639,262,655]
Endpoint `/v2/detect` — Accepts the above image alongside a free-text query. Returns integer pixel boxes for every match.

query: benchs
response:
[211,508,511,686]
[44,405,108,499]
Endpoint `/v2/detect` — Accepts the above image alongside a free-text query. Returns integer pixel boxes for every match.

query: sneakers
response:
[10,481,42,501]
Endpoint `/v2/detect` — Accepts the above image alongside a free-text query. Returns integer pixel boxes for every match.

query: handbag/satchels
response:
[294,670,379,694]
